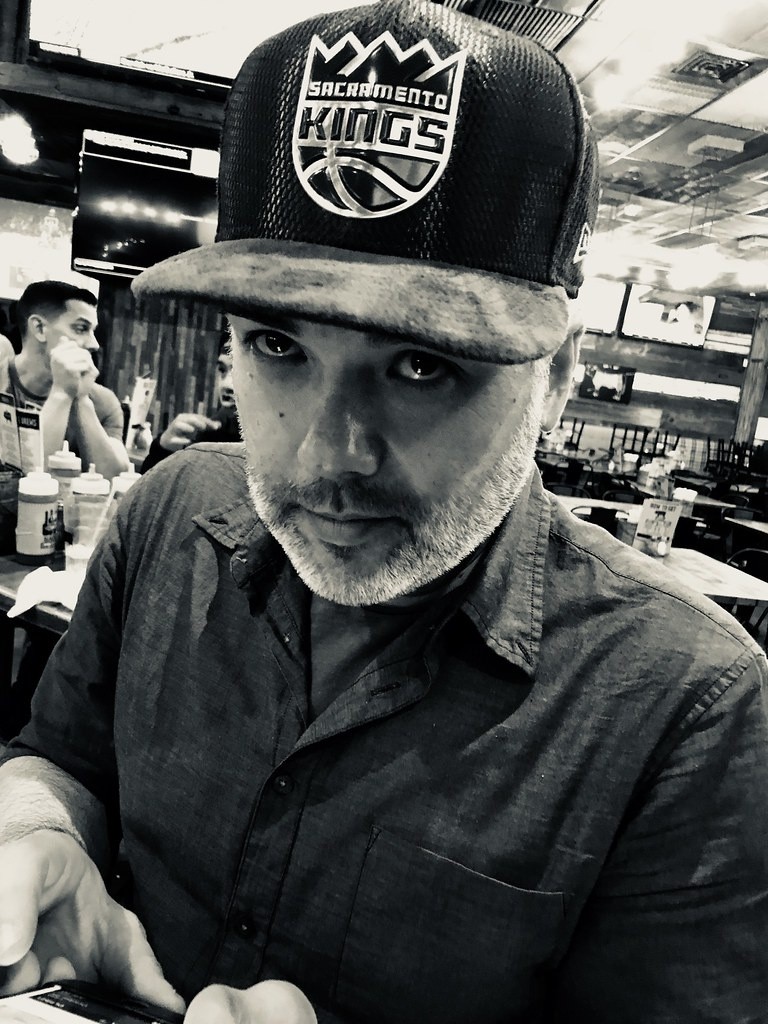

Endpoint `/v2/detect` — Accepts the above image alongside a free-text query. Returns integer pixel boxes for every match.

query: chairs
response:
[535,449,768,636]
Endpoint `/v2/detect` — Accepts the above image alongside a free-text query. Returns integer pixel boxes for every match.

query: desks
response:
[636,548,768,632]
[555,495,704,548]
[724,517,768,560]
[536,458,637,493]
[611,478,736,524]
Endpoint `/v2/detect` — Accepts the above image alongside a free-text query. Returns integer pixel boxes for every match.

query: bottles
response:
[15,467,58,567]
[47,439,82,507]
[69,463,110,548]
[92,463,142,547]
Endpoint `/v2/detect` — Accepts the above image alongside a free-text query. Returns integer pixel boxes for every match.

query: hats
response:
[129,0,600,366]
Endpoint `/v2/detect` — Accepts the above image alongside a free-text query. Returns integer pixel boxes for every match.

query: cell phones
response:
[0,979,185,1024]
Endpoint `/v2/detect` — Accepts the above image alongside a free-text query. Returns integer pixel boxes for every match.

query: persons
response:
[140,333,244,476]
[0,279,130,481]
[0,0,768,1024]
